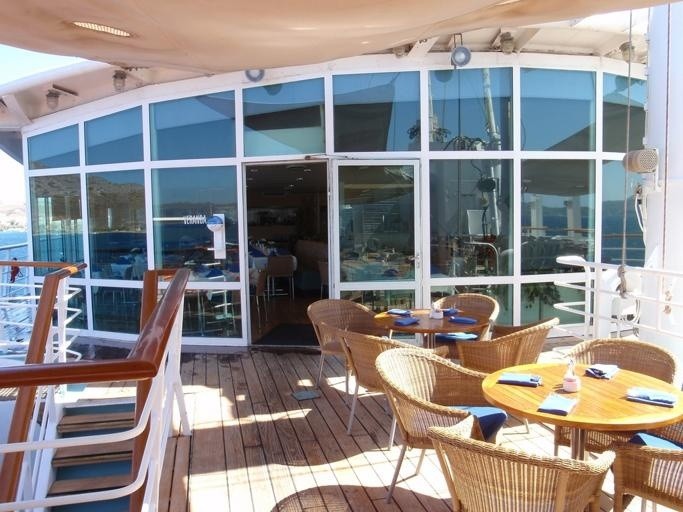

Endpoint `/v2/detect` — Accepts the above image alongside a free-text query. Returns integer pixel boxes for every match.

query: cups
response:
[562,376,580,392]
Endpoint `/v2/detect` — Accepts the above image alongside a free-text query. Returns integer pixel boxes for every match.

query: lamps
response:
[246,68,265,83]
[606,41,635,64]
[112,68,144,92]
[46,82,79,109]
[448,32,472,70]
[488,28,515,56]
